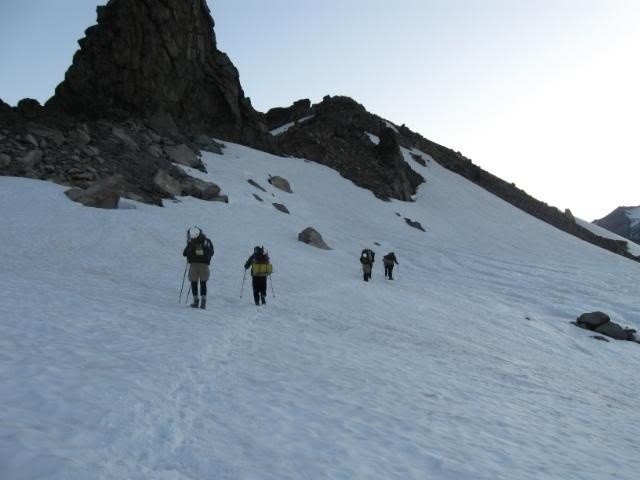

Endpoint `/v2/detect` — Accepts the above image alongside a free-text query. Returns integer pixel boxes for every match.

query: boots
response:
[190,295,207,309]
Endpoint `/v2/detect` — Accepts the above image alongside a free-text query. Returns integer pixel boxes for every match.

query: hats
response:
[189,226,201,240]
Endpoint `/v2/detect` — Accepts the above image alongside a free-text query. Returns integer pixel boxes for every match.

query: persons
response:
[244,245,272,307]
[382,251,399,281]
[361,247,375,282]
[183,225,215,310]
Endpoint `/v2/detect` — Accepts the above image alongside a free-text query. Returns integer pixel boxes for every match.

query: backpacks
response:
[251,252,271,278]
